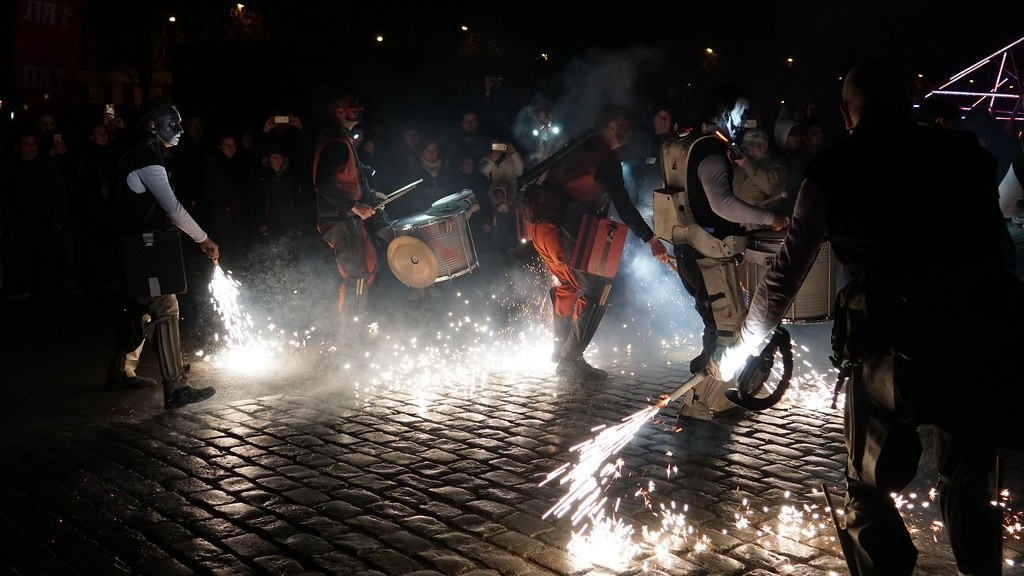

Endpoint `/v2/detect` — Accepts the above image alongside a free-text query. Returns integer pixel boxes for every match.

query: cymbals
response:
[426,201,471,216]
[387,236,438,289]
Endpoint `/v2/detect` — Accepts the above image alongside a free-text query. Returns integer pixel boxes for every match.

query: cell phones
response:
[492,143,506,150]
[275,117,290,123]
[54,134,61,145]
[106,104,115,120]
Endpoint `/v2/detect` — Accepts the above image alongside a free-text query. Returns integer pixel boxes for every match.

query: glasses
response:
[721,109,732,121]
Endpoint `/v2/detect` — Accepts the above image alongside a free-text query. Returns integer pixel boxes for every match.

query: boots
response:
[549,285,577,359]
[558,296,608,382]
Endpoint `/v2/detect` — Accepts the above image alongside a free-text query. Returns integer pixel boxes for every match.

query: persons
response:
[525,107,669,379]
[2,45,1023,428]
[706,61,1023,573]
[104,103,221,412]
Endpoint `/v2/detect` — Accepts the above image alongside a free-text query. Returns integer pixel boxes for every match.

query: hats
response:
[773,116,802,148]
[741,128,768,147]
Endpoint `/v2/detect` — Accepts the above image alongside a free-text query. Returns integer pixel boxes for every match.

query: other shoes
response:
[101,371,158,393]
[162,384,215,410]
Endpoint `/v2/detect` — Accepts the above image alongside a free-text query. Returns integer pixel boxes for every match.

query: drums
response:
[390,207,480,285]
[744,228,841,327]
[431,188,476,206]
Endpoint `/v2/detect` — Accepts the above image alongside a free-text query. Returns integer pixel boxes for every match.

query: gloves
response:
[705,345,747,383]
